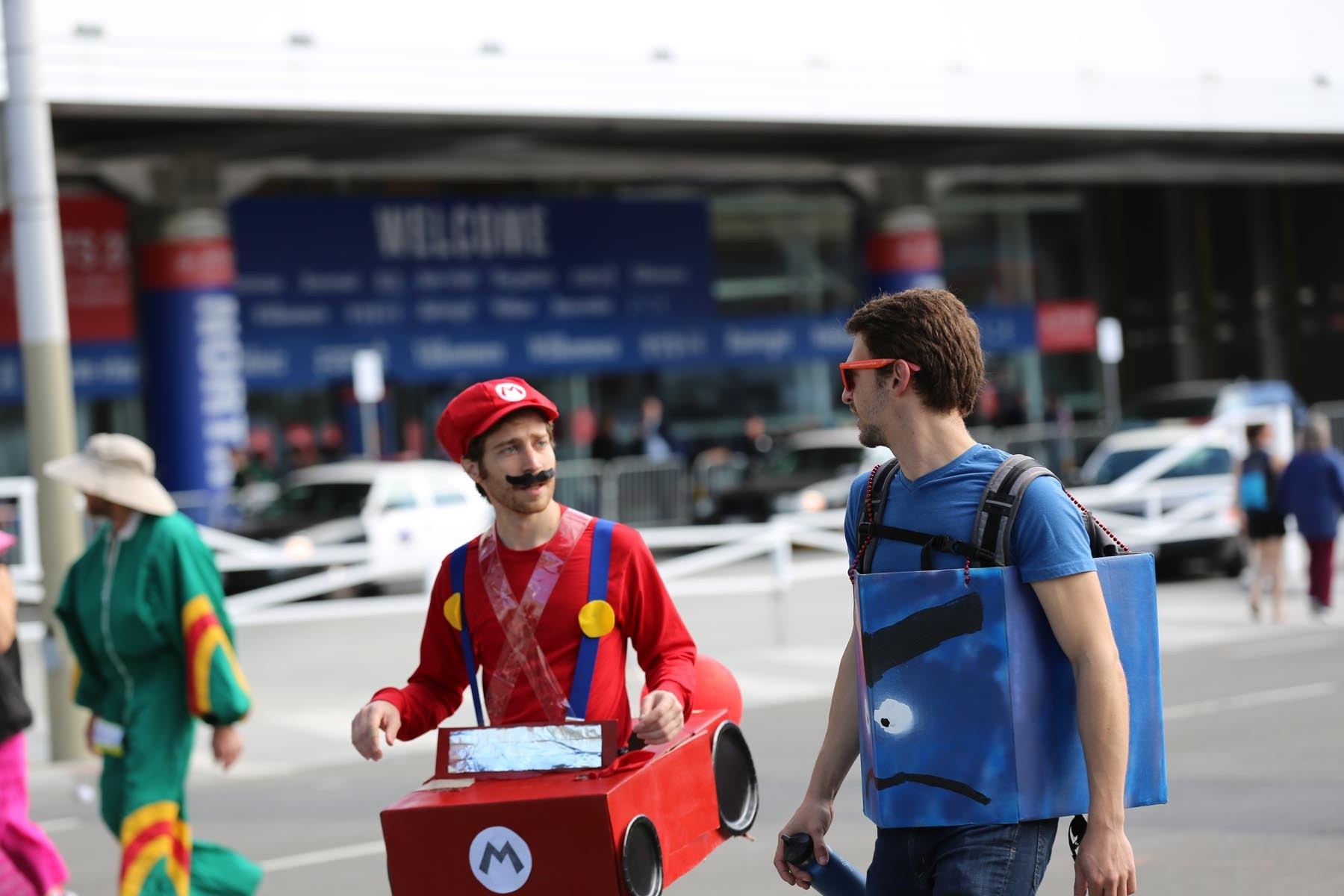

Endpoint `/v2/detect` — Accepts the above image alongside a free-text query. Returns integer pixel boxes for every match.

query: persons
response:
[0,521,72,896]
[978,360,1026,426]
[591,395,767,523]
[349,376,697,896]
[1278,413,1344,616]
[40,432,266,896]
[771,286,1139,896]
[1231,416,1285,626]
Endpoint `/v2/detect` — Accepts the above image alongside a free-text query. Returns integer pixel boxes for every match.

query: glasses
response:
[839,359,921,392]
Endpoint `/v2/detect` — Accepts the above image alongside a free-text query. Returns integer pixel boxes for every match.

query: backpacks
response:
[860,455,1132,575]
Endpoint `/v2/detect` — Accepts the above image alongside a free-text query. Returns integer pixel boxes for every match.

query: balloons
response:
[638,656,743,746]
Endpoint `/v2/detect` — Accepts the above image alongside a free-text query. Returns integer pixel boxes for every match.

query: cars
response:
[1074,423,1251,580]
[1146,377,1314,467]
[722,431,894,550]
[247,459,499,601]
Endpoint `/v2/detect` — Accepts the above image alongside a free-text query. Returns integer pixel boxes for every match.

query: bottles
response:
[781,832,867,896]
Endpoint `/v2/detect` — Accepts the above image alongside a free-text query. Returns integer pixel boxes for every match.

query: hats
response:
[44,433,179,516]
[436,376,560,464]
